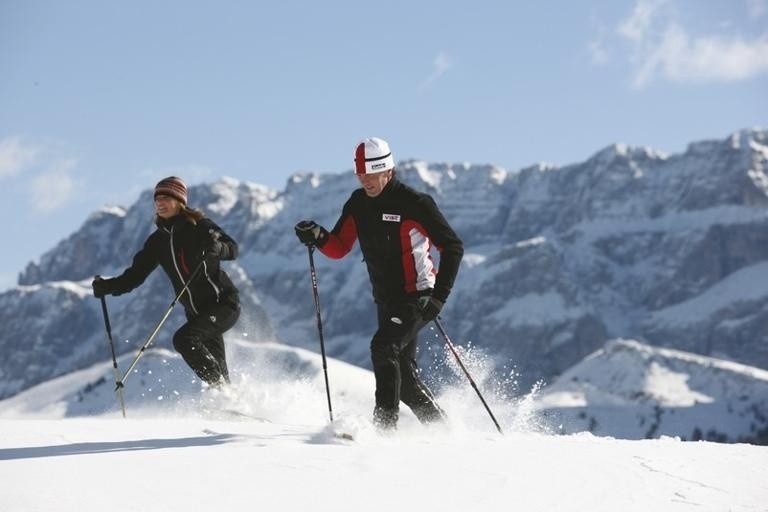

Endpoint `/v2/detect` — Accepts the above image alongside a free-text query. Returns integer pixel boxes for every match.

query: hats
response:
[354,138,394,176]
[153,176,188,205]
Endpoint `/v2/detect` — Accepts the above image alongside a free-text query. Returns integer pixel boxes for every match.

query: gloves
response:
[294,219,321,244]
[200,238,222,260]
[418,295,444,322]
[93,278,107,299]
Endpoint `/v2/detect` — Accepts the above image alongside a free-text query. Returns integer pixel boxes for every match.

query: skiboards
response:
[196,401,317,438]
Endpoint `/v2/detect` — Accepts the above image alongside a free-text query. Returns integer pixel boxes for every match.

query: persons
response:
[92,175,241,395]
[293,137,464,434]
